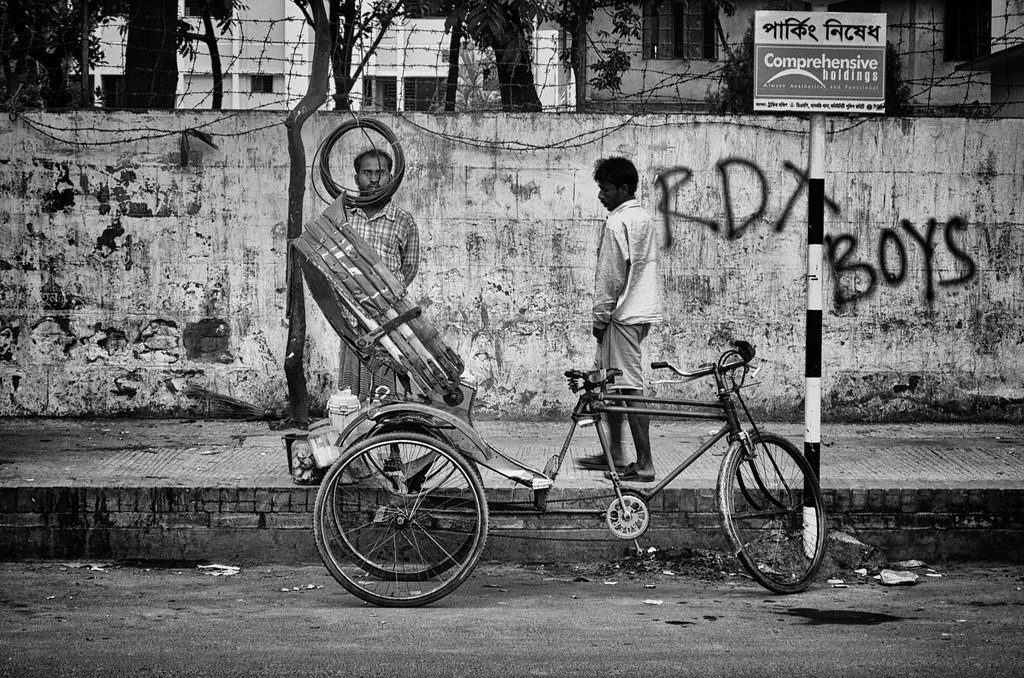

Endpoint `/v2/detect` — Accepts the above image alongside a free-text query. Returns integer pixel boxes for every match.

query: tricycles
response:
[289,190,828,607]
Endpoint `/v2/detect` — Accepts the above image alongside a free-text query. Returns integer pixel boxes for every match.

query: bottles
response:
[327,388,362,439]
[291,432,326,485]
[308,418,345,470]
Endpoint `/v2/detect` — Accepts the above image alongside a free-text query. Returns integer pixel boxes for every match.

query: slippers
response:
[576,455,625,471]
[604,461,656,481]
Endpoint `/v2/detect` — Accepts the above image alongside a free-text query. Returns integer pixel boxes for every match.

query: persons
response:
[315,149,411,472]
[573,155,668,484]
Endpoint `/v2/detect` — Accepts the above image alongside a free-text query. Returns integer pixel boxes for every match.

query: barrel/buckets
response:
[280,434,307,476]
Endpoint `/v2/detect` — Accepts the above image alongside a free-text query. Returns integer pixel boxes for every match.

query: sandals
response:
[387,457,405,472]
